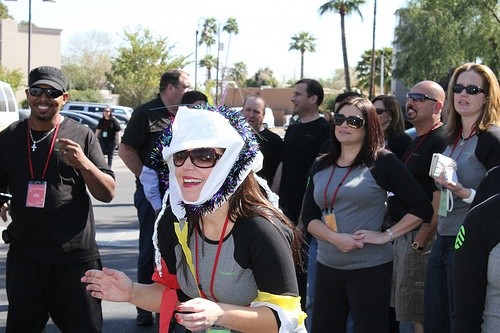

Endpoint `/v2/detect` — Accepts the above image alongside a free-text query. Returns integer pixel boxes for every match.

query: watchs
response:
[412,241,423,251]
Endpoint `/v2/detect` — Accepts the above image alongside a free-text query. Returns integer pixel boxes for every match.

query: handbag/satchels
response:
[429,153,457,212]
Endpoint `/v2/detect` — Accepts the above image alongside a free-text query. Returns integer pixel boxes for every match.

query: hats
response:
[28,65,66,92]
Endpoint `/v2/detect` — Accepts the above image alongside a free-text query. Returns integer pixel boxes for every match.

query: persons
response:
[388,80,449,333]
[140,91,208,218]
[118,70,192,325]
[95,108,122,168]
[301,95,434,333]
[373,95,413,161]
[241,95,283,187]
[271,78,333,312]
[324,111,332,121]
[80,106,308,333]
[421,62,500,333]
[0,66,117,333]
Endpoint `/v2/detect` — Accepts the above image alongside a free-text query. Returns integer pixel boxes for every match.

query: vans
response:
[211,81,239,95]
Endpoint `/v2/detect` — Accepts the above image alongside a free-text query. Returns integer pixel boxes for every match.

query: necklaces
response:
[30,126,56,151]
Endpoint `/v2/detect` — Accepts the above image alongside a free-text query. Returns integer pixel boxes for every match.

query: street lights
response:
[195,17,211,92]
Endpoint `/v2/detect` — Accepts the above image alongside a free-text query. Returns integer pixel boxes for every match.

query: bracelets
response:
[386,229,394,242]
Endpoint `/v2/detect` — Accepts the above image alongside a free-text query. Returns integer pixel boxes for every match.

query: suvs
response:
[59,102,134,150]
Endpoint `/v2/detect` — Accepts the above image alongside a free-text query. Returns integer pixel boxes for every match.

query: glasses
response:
[332,113,366,129]
[453,84,486,95]
[375,107,388,115]
[172,147,223,168]
[29,87,65,99]
[405,92,437,103]
